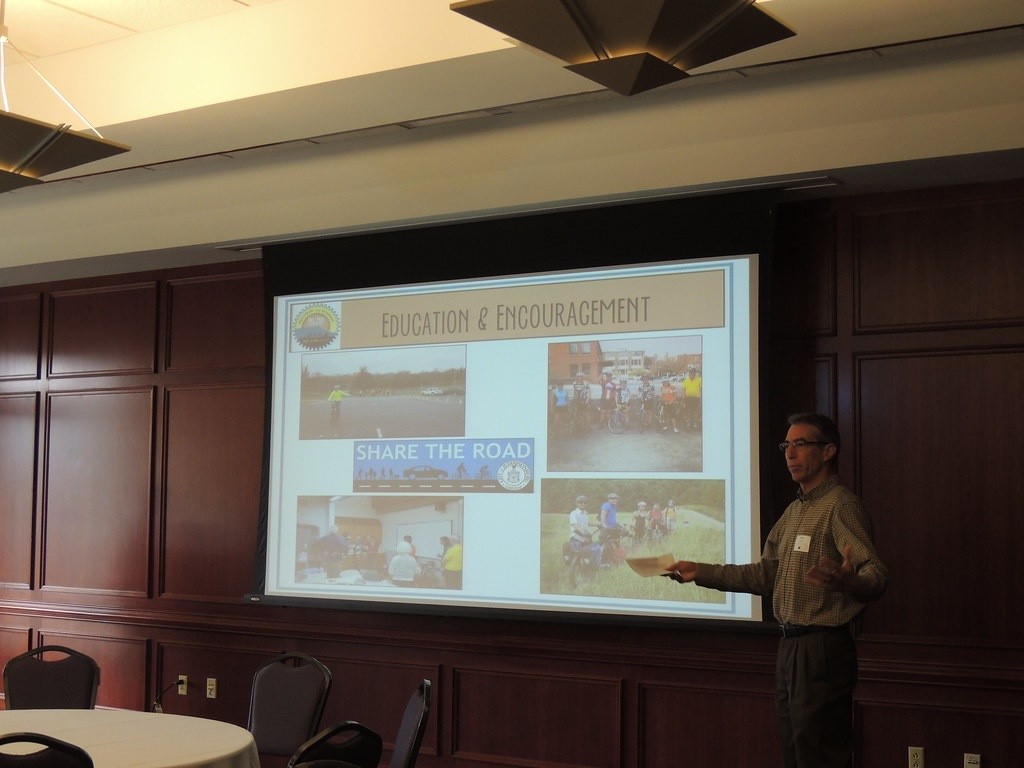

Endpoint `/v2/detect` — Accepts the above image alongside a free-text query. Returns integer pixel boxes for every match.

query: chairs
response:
[242,646,332,766]
[0,733,94,768]
[287,720,385,768]
[391,678,434,768]
[2,645,103,709]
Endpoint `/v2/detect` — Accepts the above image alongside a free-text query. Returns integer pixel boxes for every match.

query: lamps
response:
[1,1,131,200]
[451,1,799,100]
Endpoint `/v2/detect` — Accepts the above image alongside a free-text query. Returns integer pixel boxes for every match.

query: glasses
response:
[779,438,830,453]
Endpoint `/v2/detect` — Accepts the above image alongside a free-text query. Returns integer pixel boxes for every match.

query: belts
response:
[776,625,849,638]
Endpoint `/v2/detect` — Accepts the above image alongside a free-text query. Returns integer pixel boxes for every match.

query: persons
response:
[328,383,348,425]
[660,414,903,768]
[547,358,702,440]
[351,457,497,484]
[296,495,462,595]
[565,494,596,594]
[343,386,395,399]
[628,492,678,551]
[599,492,621,561]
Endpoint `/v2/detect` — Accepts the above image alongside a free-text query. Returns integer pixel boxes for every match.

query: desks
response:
[1,704,260,768]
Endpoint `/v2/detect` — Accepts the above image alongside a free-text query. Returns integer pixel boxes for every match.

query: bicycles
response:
[563,517,671,592]
[328,399,341,421]
[549,394,703,435]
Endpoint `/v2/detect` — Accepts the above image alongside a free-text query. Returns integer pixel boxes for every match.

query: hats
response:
[637,502,647,506]
[608,493,618,498]
[576,496,588,502]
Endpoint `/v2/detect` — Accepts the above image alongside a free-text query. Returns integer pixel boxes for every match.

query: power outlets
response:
[908,747,924,768]
[963,753,980,768]
[206,678,217,699]
[177,674,188,695]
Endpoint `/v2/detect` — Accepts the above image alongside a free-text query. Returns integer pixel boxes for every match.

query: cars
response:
[423,387,443,396]
[444,386,465,396]
